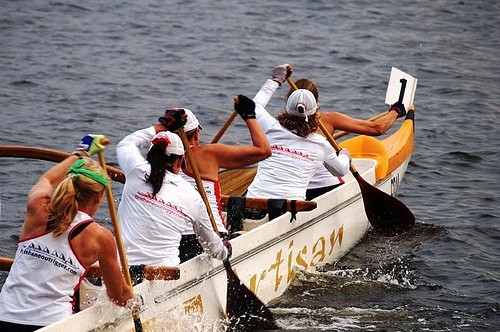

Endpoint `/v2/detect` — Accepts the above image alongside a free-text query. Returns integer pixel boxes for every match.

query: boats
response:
[0,103,415,332]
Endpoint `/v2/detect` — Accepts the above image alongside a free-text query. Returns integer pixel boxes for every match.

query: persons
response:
[168,95,273,264]
[277,78,406,202]
[0,133,144,332]
[114,108,232,285]
[244,64,351,222]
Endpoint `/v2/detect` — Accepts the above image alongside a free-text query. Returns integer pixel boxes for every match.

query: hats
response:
[148,131,188,156]
[286,89,319,121]
[171,107,203,134]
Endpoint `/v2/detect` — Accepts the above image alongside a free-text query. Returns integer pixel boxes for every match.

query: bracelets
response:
[72,151,83,160]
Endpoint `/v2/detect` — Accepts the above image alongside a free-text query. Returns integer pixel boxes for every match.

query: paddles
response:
[0,256,180,282]
[287,76,416,235]
[97,139,145,332]
[174,123,279,330]
[0,144,318,214]
[211,95,245,143]
[329,106,397,142]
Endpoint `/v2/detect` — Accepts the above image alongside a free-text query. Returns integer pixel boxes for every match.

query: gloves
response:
[271,63,289,86]
[337,147,352,161]
[234,94,257,121]
[389,102,406,118]
[223,241,232,259]
[158,107,188,130]
[74,134,106,157]
